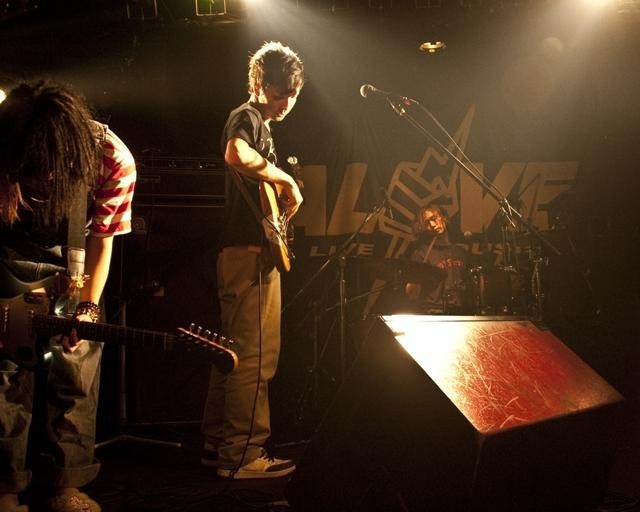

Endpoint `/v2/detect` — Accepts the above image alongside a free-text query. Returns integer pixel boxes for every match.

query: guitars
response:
[260,156,304,273]
[1,265,238,375]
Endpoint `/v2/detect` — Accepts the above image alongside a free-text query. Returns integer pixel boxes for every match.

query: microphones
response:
[360,84,418,105]
[380,186,394,220]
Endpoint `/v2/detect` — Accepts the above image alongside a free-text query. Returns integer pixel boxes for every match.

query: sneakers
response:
[48,486,102,512]
[200,442,221,470]
[0,491,31,512]
[216,449,297,480]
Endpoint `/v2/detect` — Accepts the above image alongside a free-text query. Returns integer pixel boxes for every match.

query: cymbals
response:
[359,257,447,284]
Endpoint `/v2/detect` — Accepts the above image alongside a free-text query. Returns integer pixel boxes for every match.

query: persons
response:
[202,41,304,480]
[0,81,136,512]
[407,206,488,314]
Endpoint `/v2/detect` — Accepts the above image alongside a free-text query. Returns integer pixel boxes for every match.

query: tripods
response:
[94,303,182,449]
[297,302,337,414]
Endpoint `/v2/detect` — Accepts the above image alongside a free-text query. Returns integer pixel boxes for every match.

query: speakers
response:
[117,168,228,431]
[284,315,627,511]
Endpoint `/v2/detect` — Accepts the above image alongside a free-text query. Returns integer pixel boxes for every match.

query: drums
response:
[462,265,511,316]
